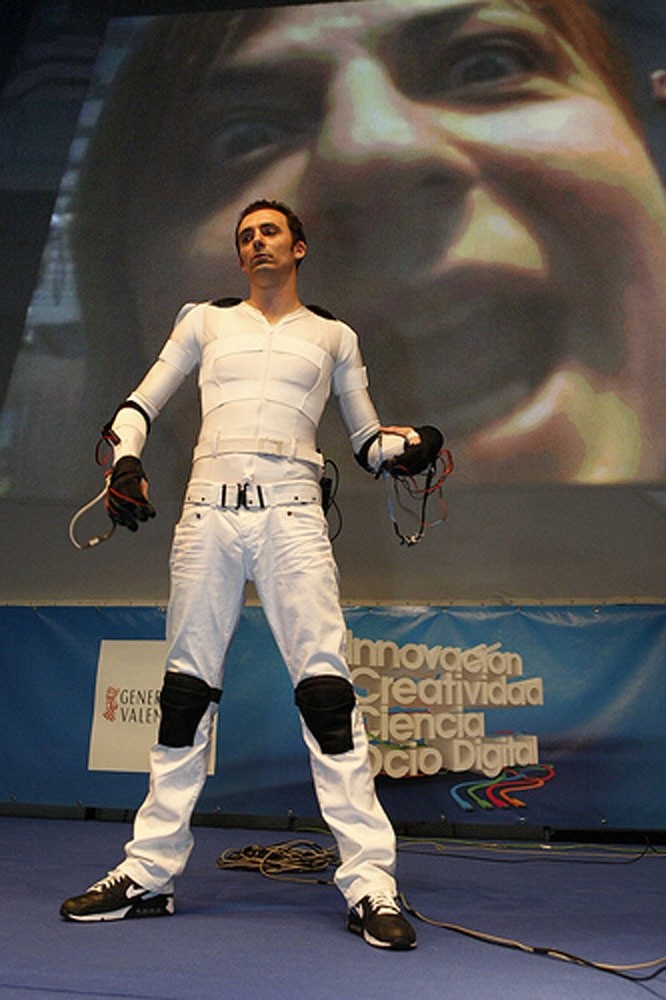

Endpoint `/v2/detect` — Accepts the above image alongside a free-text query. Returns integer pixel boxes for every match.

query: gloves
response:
[354,426,443,477]
[105,456,156,532]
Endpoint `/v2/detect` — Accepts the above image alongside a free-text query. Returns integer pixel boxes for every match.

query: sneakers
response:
[57,871,175,921]
[348,895,418,950]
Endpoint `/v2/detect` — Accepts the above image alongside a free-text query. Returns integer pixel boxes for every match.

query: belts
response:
[186,483,321,511]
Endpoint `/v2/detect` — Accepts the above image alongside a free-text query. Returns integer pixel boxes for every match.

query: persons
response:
[60,199,445,953]
[75,0,666,505]
